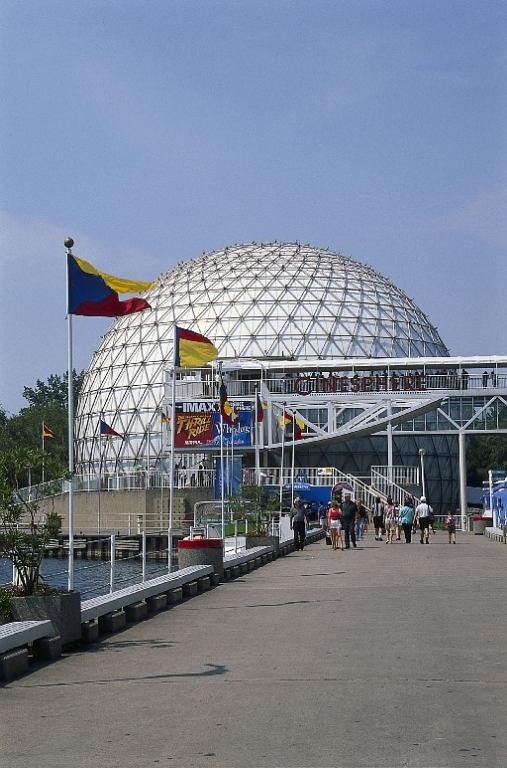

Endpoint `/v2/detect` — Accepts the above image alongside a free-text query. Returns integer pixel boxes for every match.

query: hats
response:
[421,495,427,502]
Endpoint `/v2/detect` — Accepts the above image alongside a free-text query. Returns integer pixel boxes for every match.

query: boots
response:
[420,538,429,544]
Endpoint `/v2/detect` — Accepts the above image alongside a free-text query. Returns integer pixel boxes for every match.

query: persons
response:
[481,371,498,389]
[283,368,469,392]
[133,462,155,487]
[242,465,249,485]
[289,492,435,550]
[203,368,229,393]
[445,511,456,544]
[172,459,213,486]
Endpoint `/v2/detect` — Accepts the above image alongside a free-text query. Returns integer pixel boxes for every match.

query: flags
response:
[178,326,219,369]
[101,420,126,439]
[68,253,161,317]
[213,385,305,440]
[42,424,55,438]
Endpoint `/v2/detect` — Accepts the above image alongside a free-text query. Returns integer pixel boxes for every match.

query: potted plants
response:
[224,485,282,550]
[0,446,90,648]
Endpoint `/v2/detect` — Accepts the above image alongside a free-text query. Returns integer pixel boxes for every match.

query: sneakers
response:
[296,545,304,551]
